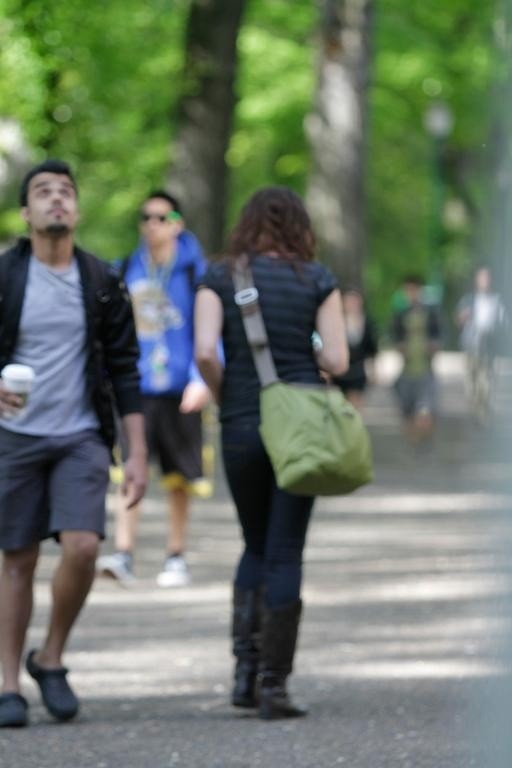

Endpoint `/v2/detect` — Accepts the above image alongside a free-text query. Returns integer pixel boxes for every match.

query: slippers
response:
[25,651,79,719]
[1,693,29,728]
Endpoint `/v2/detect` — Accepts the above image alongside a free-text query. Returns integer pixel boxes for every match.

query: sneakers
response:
[98,554,131,582]
[156,555,190,584]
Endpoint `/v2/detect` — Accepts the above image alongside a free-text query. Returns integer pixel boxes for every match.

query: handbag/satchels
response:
[258,381,374,497]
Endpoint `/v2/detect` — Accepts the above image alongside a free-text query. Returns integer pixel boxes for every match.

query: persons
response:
[391,273,440,448]
[93,188,226,586]
[450,265,505,397]
[1,161,150,729]
[192,186,349,718]
[332,284,377,410]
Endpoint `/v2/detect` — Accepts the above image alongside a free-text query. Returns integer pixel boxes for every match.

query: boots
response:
[233,581,306,718]
